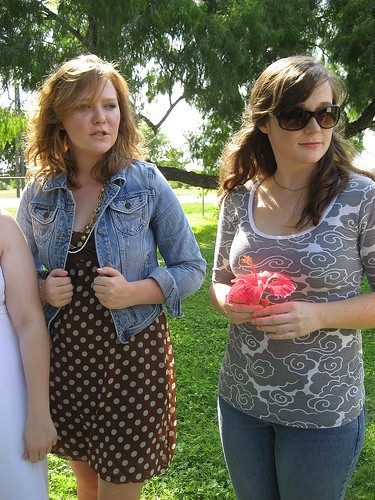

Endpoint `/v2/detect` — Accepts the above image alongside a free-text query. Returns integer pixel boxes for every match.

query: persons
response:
[205,53,375,499]
[0,211,60,500]
[18,53,209,499]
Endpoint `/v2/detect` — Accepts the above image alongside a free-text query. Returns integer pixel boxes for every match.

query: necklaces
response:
[67,176,106,250]
[272,173,308,193]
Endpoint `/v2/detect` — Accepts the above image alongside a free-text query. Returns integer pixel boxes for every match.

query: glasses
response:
[276,105,340,131]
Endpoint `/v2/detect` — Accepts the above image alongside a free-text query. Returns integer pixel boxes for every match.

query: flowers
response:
[226,256,296,307]
